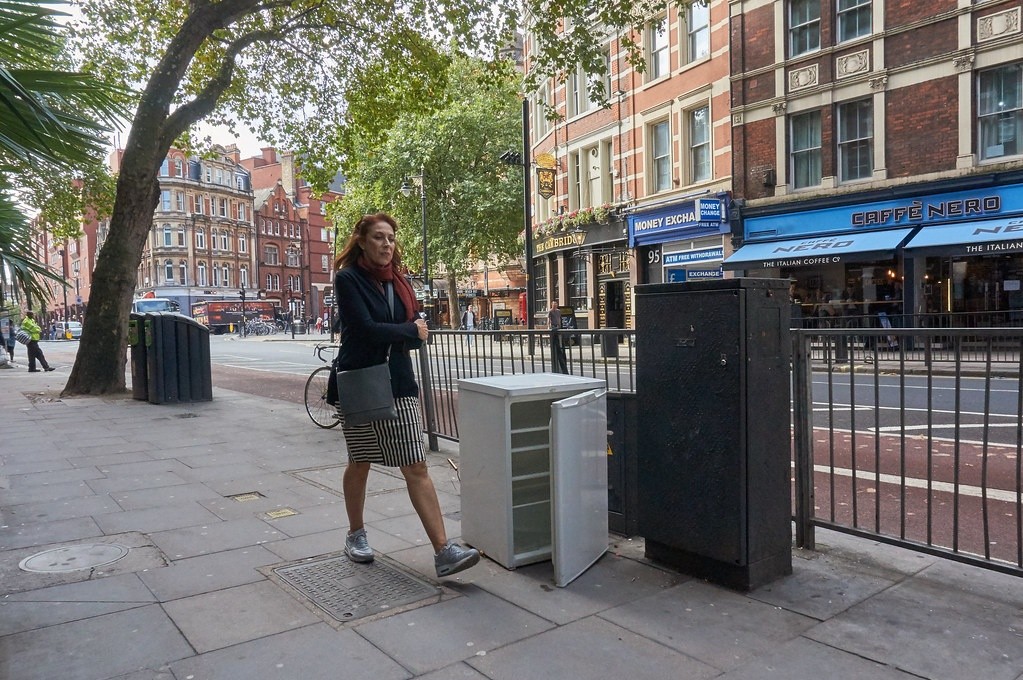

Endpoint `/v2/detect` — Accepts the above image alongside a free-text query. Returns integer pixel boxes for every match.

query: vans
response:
[54,321,83,340]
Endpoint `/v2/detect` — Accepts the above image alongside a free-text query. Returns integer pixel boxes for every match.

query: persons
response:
[809,287,826,302]
[514,317,524,326]
[926,297,938,313]
[308,314,323,332]
[789,284,802,302]
[0,319,17,362]
[42,323,63,340]
[886,281,902,301]
[547,300,562,329]
[461,304,477,347]
[843,284,861,301]
[21,311,56,372]
[326,214,481,578]
[285,311,295,334]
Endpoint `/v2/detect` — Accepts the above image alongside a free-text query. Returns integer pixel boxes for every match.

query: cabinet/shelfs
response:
[635,278,796,599]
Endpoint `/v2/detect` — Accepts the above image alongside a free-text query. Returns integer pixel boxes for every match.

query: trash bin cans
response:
[294,318,306,334]
[600,327,619,356]
[145,309,212,403]
[129,312,149,401]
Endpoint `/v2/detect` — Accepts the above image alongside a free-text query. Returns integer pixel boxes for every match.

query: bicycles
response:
[240,317,278,336]
[304,339,340,429]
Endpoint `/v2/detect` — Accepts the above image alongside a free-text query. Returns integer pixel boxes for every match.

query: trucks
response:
[191,300,282,335]
[131,298,171,313]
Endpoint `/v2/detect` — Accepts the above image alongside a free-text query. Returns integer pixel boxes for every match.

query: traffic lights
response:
[239,290,245,300]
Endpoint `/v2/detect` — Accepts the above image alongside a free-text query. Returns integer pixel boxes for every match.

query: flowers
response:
[532,201,613,238]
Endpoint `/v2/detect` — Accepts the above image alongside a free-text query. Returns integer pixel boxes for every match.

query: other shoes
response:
[471,345,474,347]
[28,369,41,372]
[44,367,55,372]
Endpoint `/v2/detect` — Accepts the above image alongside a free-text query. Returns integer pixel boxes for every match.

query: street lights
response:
[400,162,434,346]
[58,250,69,338]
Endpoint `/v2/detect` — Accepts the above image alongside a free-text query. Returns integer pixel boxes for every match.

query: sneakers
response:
[344,527,374,562]
[432,541,481,577]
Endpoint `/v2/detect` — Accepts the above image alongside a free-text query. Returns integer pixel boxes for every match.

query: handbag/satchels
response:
[458,325,465,330]
[336,362,401,428]
[15,330,31,345]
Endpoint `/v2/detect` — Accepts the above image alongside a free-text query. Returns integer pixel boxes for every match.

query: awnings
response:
[721,227,915,272]
[904,215,1023,258]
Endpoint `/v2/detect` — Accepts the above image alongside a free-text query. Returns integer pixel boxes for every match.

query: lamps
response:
[535,233,540,239]
[613,90,625,96]
[546,230,553,236]
